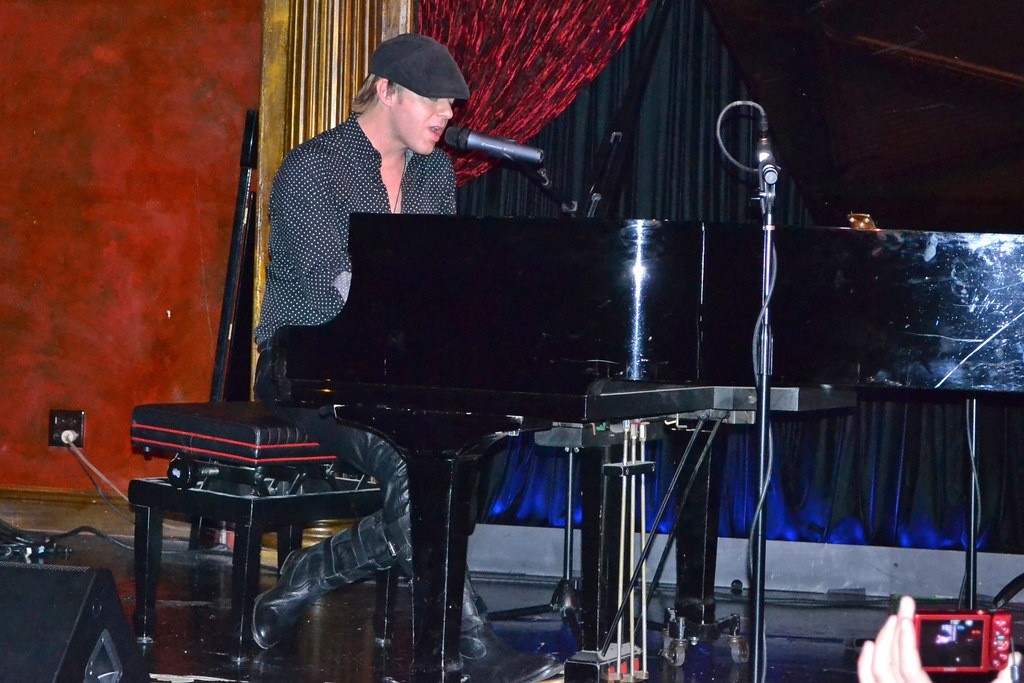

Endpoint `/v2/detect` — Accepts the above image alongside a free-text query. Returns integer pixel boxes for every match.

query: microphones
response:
[756,113,773,163]
[445,126,544,163]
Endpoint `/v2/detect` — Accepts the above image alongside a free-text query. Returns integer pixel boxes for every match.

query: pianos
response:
[274,211,1021,683]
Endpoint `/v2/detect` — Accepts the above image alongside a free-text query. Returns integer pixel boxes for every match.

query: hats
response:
[369,34,470,100]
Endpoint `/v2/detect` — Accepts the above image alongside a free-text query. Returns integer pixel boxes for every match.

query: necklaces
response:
[393,153,407,212]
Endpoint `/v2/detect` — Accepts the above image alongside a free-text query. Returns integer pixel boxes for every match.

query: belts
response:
[257,337,272,352]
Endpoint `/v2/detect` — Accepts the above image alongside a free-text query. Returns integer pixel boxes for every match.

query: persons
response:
[253,34,565,683]
[858,596,1023,683]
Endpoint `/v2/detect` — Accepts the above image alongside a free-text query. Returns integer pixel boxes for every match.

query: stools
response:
[127,399,402,663]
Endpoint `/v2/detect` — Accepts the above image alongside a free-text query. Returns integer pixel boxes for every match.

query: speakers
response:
[0,561,151,683]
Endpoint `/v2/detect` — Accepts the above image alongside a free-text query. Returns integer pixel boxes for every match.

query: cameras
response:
[912,608,1012,671]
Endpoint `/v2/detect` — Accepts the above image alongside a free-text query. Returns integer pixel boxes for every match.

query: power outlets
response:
[48,409,85,447]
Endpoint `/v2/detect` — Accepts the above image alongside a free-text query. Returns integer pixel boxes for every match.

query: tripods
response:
[482,160,669,650]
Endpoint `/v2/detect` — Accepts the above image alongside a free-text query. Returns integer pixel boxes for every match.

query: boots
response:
[385,513,563,683]
[250,507,385,650]
